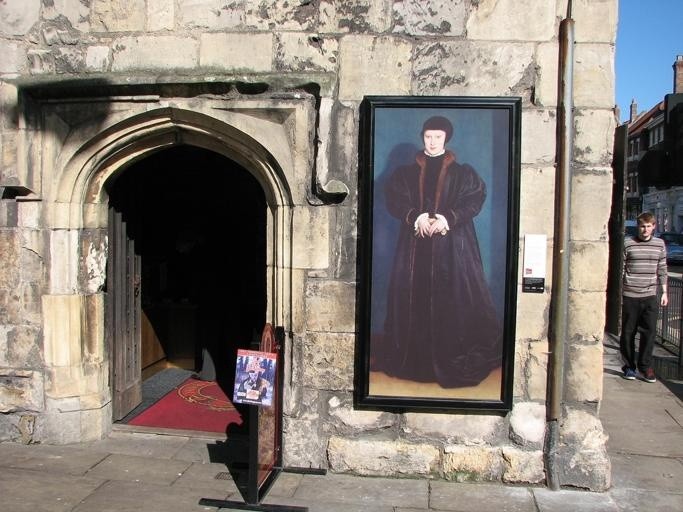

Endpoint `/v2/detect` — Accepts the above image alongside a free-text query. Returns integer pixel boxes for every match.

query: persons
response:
[237,370,267,404]
[618,212,669,382]
[370,114,503,387]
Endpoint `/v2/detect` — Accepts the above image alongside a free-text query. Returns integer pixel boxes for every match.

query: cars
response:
[655,231,683,265]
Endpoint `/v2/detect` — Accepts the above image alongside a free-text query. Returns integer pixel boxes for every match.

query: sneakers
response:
[623,367,656,382]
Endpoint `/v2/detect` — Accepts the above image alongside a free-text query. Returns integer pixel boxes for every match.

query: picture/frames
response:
[350,93,523,415]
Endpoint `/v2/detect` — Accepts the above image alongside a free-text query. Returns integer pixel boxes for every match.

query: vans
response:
[624,219,637,237]
